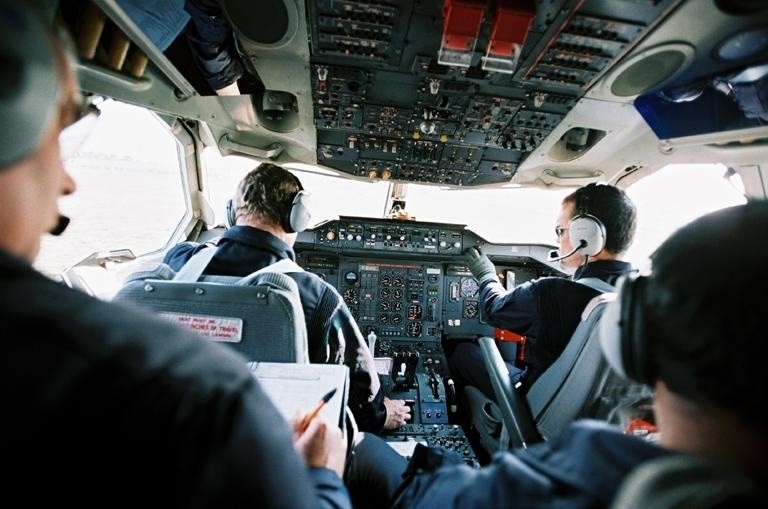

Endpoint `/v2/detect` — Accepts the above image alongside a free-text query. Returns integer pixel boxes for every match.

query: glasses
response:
[555,225,568,238]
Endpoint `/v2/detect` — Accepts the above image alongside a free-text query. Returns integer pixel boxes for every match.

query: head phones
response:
[598,203,747,388]
[567,181,607,257]
[0,0,61,170]
[222,161,313,234]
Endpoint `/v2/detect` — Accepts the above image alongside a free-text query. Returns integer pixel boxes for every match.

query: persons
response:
[449,182,643,453]
[346,200,767,509]
[147,159,413,429]
[2,3,351,509]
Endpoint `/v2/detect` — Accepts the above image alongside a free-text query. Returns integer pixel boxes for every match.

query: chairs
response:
[110,262,310,363]
[459,292,653,460]
[609,454,768,509]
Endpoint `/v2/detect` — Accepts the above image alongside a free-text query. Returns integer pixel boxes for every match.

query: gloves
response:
[464,245,495,279]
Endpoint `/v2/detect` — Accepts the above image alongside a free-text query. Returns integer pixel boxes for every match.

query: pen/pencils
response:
[296,389,336,437]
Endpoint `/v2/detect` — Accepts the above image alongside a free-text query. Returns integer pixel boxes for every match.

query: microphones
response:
[547,239,588,263]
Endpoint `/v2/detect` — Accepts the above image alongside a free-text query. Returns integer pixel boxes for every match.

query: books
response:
[233,359,344,431]
